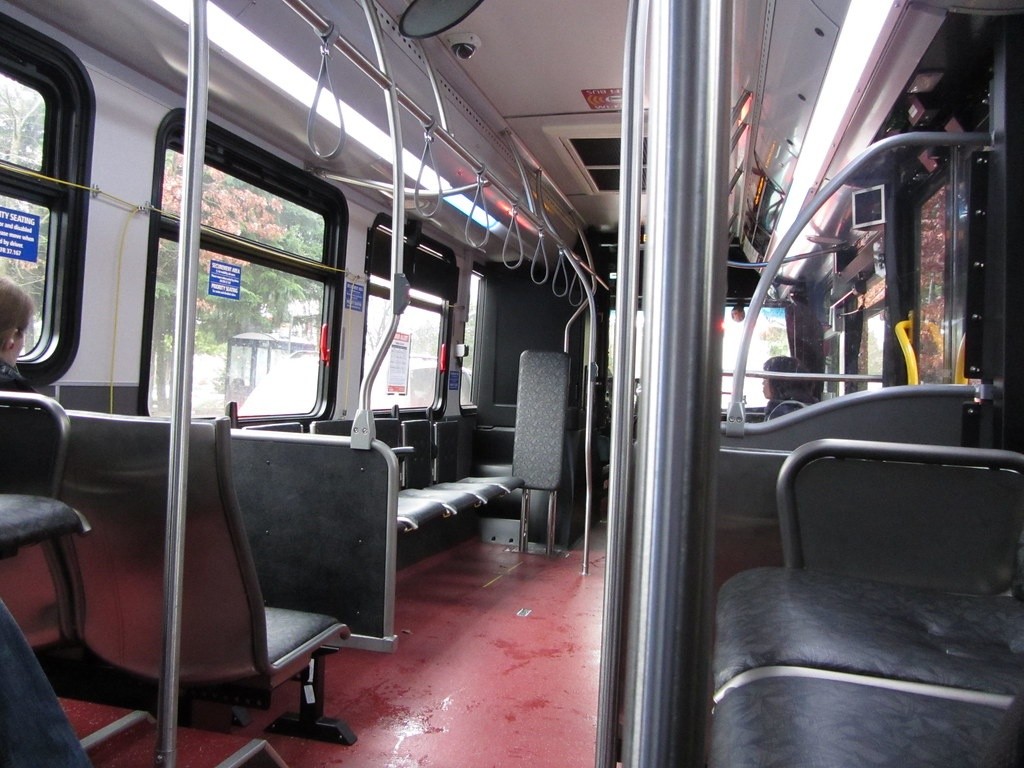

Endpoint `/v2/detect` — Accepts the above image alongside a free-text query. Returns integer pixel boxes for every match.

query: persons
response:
[764,357,819,427]
[0,277,43,393]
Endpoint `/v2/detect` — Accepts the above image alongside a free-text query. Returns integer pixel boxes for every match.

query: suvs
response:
[235,348,472,426]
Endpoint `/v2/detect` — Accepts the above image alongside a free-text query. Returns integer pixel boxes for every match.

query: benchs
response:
[714,383,1024,768]
[0,391,528,746]
